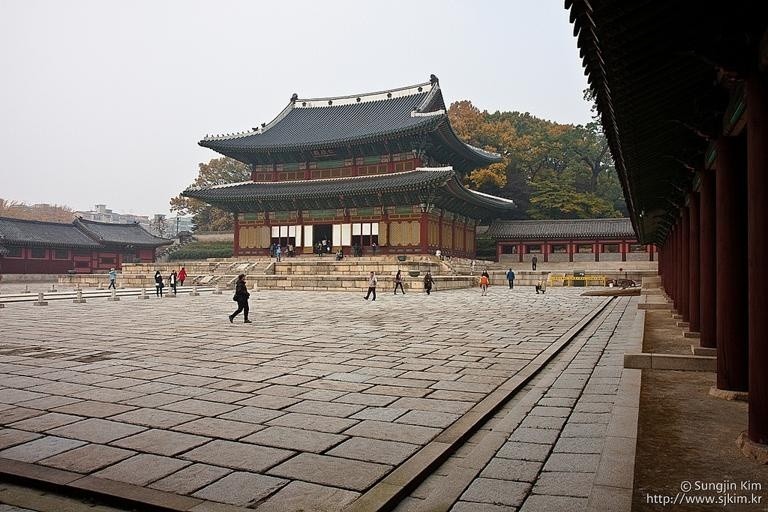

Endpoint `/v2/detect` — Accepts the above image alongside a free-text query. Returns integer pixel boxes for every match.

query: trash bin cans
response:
[574,270,585,287]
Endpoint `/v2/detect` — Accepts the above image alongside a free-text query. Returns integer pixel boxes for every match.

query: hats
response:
[237,273,246,279]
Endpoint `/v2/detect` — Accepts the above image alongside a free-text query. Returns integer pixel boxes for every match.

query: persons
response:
[270,236,343,263]
[393,269,406,295]
[531,254,537,270]
[481,268,489,278]
[168,269,177,295]
[371,242,376,255]
[478,273,489,296]
[106,267,116,290]
[535,278,546,294]
[423,271,434,294]
[363,271,377,301]
[506,268,515,289]
[177,267,187,286]
[154,271,163,298]
[228,273,252,324]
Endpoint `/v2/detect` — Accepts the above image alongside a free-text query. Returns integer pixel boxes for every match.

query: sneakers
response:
[242,319,252,324]
[228,315,234,324]
